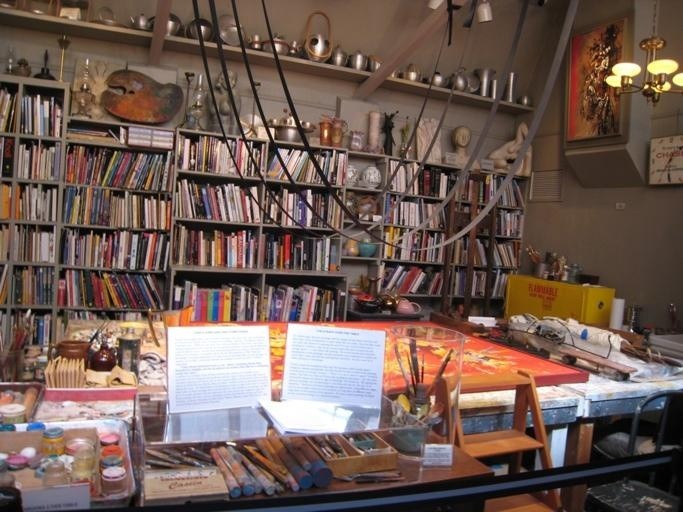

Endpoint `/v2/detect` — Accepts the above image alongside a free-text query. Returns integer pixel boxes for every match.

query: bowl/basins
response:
[272,126,312,143]
[186,25,215,45]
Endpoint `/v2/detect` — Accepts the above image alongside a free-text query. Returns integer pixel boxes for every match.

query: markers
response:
[267,426,333,490]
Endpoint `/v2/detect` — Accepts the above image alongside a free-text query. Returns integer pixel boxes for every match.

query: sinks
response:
[649,332,682,357]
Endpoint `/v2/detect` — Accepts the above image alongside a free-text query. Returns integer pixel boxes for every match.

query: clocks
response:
[647,134,683,188]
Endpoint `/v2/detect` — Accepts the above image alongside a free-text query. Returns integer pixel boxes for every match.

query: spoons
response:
[397,394,443,426]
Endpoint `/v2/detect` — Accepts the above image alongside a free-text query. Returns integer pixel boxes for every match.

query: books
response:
[1,89,348,356]
[383,159,526,298]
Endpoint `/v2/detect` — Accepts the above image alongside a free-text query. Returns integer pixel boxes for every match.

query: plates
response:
[217,14,245,47]
[357,196,380,221]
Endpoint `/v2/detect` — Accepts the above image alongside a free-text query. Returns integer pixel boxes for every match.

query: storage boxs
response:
[504,273,616,328]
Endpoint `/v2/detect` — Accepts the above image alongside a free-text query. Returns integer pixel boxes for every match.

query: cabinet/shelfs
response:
[0,72,530,349]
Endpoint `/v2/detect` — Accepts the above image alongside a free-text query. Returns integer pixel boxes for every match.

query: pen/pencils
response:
[210,437,300,499]
[281,436,312,471]
[309,434,350,459]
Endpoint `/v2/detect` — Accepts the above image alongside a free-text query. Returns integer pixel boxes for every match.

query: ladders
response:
[432,367,564,512]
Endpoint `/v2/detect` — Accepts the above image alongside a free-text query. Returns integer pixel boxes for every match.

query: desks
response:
[0,300,683,512]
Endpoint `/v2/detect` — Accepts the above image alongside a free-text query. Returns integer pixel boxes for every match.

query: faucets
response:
[667,302,677,328]
[608,297,625,330]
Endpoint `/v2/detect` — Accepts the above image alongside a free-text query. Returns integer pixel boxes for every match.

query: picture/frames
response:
[563,16,627,146]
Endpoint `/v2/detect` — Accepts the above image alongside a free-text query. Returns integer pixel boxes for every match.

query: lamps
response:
[475,0,493,23]
[427,0,444,10]
[607,1,683,107]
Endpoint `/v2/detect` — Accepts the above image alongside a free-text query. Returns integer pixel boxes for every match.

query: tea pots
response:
[129,12,153,31]
[354,294,421,316]
[165,16,181,36]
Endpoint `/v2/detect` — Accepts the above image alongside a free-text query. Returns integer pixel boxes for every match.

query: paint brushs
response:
[89,318,112,343]
[395,328,454,398]
[526,244,540,265]
[145,446,217,468]
[0,307,36,383]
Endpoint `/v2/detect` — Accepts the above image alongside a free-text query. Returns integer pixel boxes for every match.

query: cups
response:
[48,340,91,372]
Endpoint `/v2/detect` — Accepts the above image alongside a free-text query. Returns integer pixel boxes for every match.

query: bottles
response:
[39,427,128,496]
[608,298,625,331]
[560,264,569,281]
[90,327,140,376]
[279,109,293,126]
